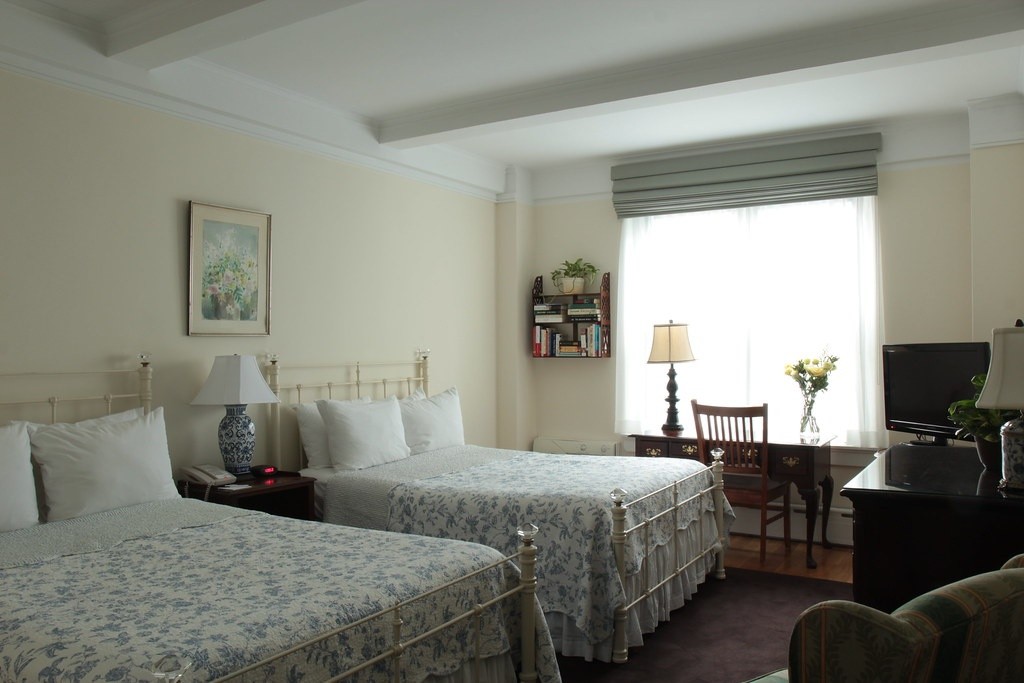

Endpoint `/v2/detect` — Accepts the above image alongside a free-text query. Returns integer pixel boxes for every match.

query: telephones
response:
[175,463,238,486]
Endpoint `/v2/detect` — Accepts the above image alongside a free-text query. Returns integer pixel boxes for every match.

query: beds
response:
[266,349,737,663]
[0,348,565,683]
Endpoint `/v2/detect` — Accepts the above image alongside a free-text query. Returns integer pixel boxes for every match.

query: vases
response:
[798,400,820,444]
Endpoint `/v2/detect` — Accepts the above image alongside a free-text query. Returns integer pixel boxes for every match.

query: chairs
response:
[692,397,792,562]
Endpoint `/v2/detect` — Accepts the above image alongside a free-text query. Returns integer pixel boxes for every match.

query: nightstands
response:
[179,466,317,522]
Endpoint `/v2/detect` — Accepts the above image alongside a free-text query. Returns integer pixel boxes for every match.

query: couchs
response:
[741,554,1024,683]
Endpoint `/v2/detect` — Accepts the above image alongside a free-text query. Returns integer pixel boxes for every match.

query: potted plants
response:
[539,258,598,302]
[948,370,1021,474]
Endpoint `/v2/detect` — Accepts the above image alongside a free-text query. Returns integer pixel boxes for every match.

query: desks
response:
[628,428,835,570]
[840,442,1024,607]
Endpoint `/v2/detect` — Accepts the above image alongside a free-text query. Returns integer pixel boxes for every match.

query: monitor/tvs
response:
[882,342,991,445]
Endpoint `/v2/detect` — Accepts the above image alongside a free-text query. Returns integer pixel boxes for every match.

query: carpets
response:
[554,563,854,683]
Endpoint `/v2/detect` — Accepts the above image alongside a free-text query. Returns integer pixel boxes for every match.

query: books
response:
[534,303,568,323]
[567,304,601,321]
[536,323,608,358]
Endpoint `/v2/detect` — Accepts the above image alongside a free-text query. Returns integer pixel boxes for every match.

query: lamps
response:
[975,326,1024,489]
[645,320,696,430]
[191,355,281,472]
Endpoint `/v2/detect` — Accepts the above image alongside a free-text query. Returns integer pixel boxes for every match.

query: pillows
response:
[313,395,411,472]
[28,407,182,522]
[288,396,373,470]
[395,386,464,453]
[0,421,40,530]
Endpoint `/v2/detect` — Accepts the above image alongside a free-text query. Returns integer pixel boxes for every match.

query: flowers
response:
[783,344,839,439]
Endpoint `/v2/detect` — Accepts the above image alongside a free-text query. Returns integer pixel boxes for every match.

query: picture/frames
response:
[188,201,271,339]
